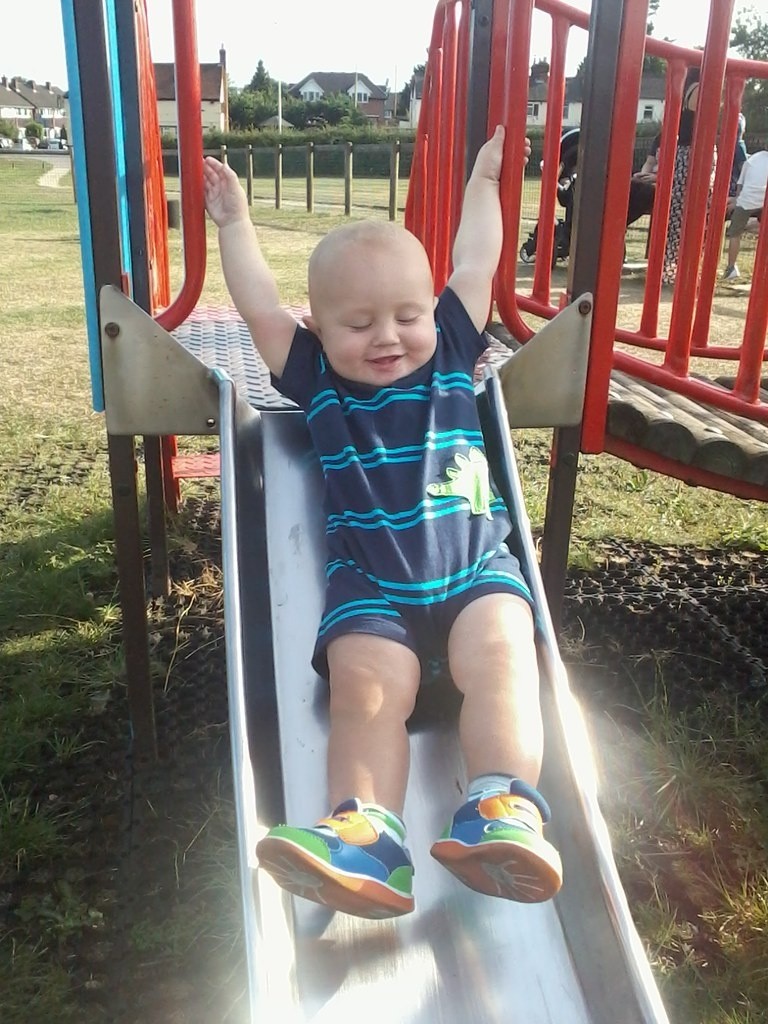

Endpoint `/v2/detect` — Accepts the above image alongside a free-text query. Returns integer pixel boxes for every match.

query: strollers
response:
[520,126,655,264]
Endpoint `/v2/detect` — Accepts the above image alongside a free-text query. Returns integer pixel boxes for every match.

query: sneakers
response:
[718,266,740,281]
[430,777,563,902]
[257,797,415,920]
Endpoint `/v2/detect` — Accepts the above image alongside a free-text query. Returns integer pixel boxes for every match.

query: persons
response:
[625,46,768,283]
[201,124,564,920]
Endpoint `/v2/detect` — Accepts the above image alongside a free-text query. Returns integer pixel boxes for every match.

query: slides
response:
[221,374,682,1024]
